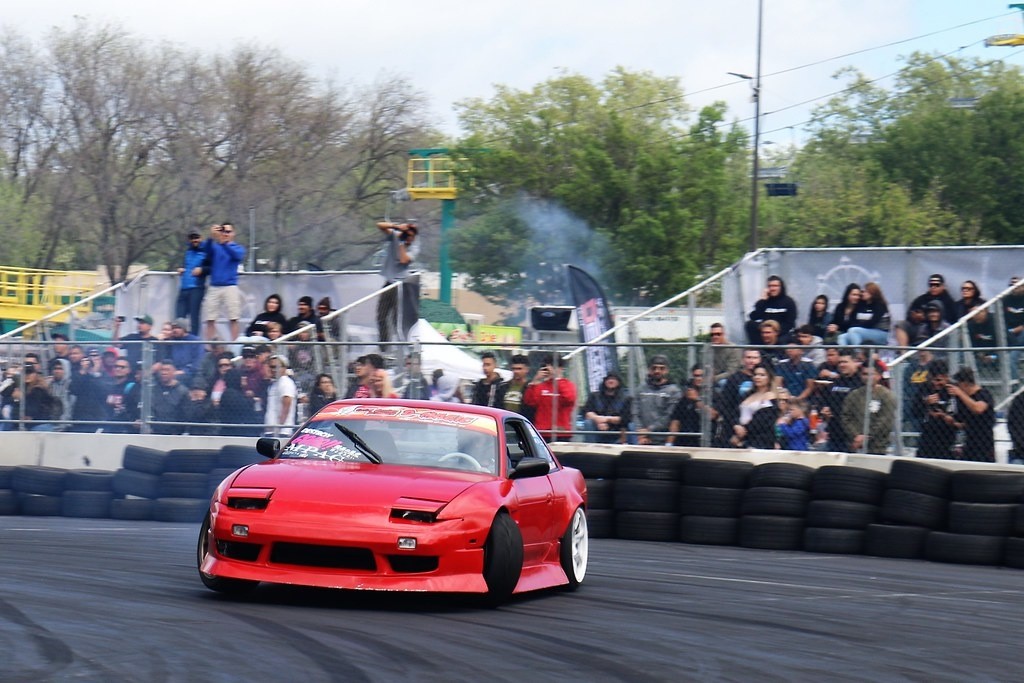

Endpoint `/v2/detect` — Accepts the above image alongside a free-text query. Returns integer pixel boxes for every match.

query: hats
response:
[242,344,257,352]
[134,314,153,325]
[318,297,331,309]
[23,363,37,373]
[171,317,186,332]
[187,227,201,236]
[191,376,207,389]
[650,354,671,365]
[299,296,312,308]
[52,333,69,341]
[929,274,944,282]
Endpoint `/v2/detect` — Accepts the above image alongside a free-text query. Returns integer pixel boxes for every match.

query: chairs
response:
[363,430,402,463]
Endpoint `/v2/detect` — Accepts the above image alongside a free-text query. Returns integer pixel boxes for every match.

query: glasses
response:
[961,287,974,291]
[114,365,127,369]
[652,366,667,371]
[270,365,285,369]
[776,398,789,403]
[242,354,255,359]
[53,366,63,371]
[861,289,868,293]
[709,332,721,337]
[223,230,233,234]
[1008,283,1020,289]
[218,363,232,369]
[929,283,940,287]
[318,307,328,312]
[189,235,200,240]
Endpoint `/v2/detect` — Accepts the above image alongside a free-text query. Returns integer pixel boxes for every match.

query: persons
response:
[175,229,212,336]
[0,273,1024,464]
[376,222,422,353]
[201,222,247,341]
[458,436,513,477]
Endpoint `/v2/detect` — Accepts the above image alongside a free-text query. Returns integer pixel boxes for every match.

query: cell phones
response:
[118,317,125,322]
[540,362,548,370]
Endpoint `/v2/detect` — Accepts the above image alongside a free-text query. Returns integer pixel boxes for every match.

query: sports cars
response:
[198,399,591,608]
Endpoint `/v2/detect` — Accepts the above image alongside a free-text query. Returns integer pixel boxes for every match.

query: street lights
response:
[726,0,764,253]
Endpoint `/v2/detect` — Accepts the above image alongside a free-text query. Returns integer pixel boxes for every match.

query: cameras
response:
[24,362,34,373]
[218,227,225,231]
[400,232,408,241]
